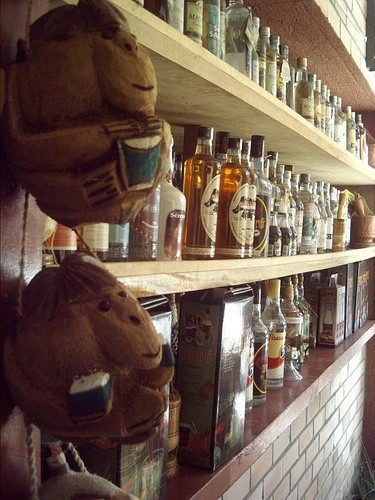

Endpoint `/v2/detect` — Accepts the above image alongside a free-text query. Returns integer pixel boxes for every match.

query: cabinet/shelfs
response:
[98,0,375,301]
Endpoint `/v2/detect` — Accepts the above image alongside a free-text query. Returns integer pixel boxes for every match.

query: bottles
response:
[43,0,375,500]
